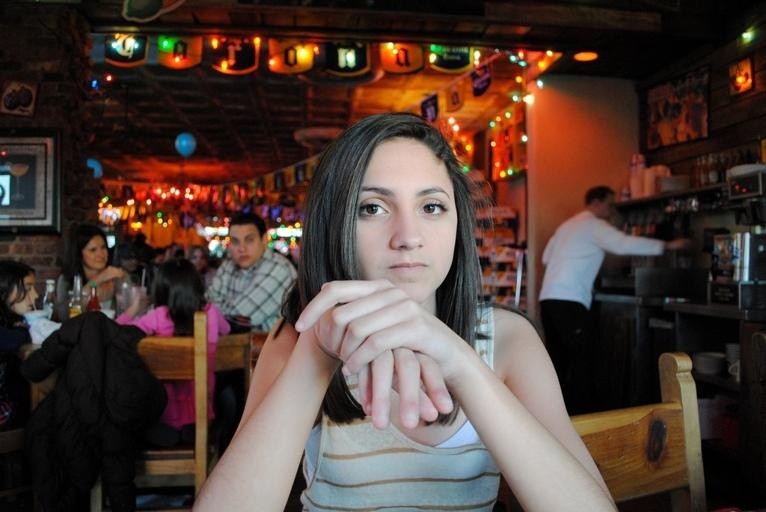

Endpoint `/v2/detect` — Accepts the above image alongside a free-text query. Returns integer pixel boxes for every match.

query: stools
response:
[0,426,28,511]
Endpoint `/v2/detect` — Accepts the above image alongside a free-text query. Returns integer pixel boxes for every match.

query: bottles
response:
[85,281,103,310]
[43,280,60,322]
[692,146,760,187]
[69,273,84,318]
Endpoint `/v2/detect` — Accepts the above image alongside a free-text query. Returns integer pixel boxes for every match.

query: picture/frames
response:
[0,125,62,234]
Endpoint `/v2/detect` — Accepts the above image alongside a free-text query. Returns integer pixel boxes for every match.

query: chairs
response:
[496,347,711,511]
[88,310,220,512]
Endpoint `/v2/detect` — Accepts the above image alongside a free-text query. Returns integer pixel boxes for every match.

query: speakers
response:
[0,80,40,116]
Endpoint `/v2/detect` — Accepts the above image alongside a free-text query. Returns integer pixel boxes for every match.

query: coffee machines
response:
[727,175,766,310]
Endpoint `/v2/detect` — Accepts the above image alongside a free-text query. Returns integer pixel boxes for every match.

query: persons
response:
[536,186,692,413]
[191,109,619,511]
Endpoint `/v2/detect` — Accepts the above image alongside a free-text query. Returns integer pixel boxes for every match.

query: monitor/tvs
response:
[639,64,711,153]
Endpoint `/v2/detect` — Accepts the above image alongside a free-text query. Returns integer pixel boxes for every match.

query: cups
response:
[26,312,49,325]
[114,281,155,318]
[620,153,672,201]
[99,310,115,319]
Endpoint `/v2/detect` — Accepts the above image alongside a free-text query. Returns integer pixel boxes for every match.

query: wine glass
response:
[7,163,30,201]
